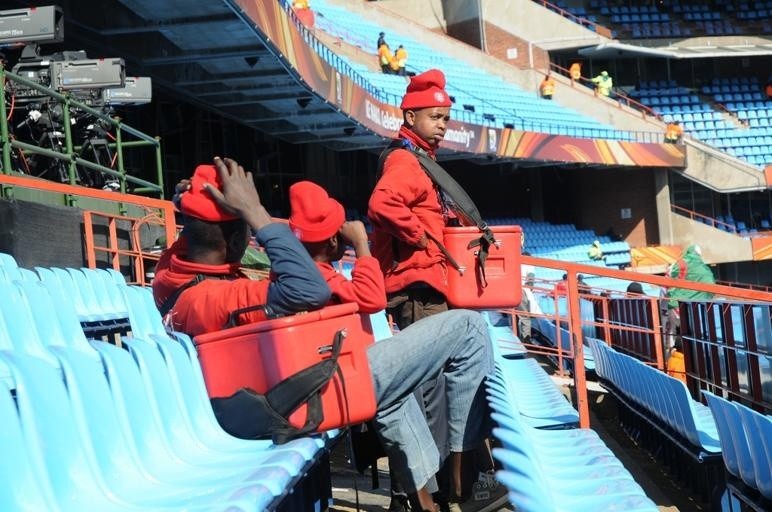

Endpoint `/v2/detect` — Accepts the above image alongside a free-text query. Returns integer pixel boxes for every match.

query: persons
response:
[521,251,535,287]
[587,239,601,261]
[151,155,510,512]
[577,274,590,294]
[591,71,612,95]
[378,32,408,77]
[569,61,583,82]
[539,75,555,101]
[556,273,568,292]
[271,180,462,512]
[368,68,463,512]
[626,281,644,295]
[664,333,686,384]
[656,243,716,347]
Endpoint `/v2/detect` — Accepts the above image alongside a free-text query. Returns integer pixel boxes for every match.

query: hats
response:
[177,165,240,222]
[287,181,347,243]
[400,69,453,109]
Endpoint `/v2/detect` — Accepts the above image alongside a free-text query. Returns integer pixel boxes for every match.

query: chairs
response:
[642,1,770,172]
[2,206,770,507]
[280,1,642,143]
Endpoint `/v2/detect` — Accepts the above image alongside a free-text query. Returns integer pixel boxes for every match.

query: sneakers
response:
[448,472,509,511]
